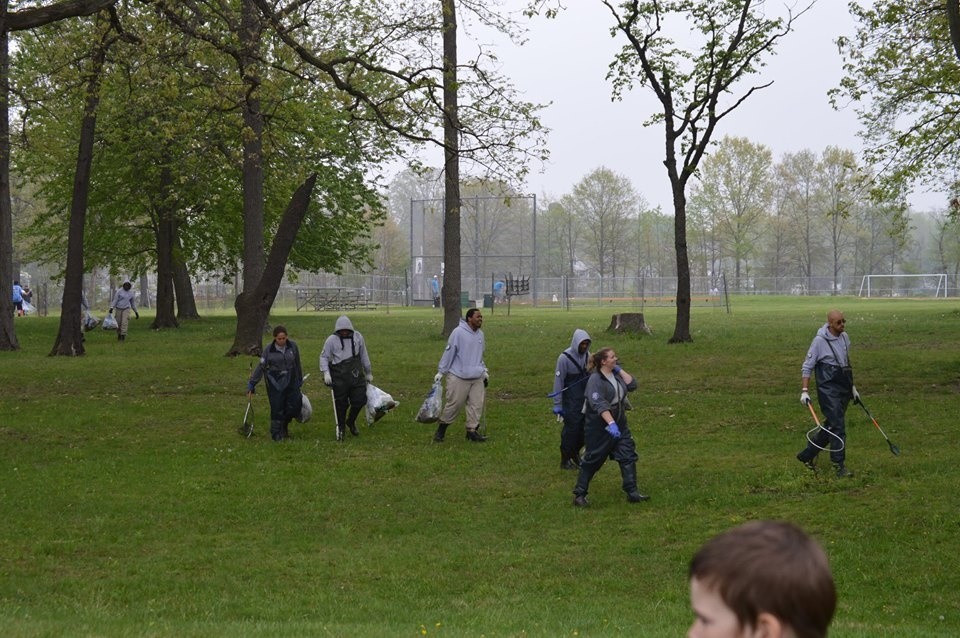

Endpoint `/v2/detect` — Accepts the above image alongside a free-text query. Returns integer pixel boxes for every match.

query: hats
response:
[25,284,29,290]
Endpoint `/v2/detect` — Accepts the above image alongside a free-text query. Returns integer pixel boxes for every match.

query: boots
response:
[432,422,449,442]
[338,416,347,440]
[572,467,596,508]
[346,407,361,436]
[465,430,487,443]
[620,462,648,503]
[573,450,584,467]
[559,452,577,470]
[271,420,291,442]
[117,334,124,341]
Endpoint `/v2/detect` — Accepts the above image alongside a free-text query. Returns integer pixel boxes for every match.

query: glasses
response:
[832,320,847,324]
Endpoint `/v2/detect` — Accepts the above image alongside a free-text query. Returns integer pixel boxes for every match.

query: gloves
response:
[613,365,621,374]
[366,374,374,384]
[799,388,812,408]
[484,368,490,387]
[434,373,443,383]
[608,422,621,439]
[851,384,860,405]
[323,372,333,386]
[136,312,139,319]
[109,308,113,313]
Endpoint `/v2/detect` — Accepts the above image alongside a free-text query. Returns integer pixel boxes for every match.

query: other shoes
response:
[796,448,817,473]
[832,462,853,479]
[18,313,20,317]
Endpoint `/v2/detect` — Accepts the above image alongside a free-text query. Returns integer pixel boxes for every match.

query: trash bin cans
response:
[484,294,493,307]
[460,291,469,308]
[468,300,476,308]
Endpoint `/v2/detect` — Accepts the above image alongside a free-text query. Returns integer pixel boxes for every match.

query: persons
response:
[797,310,860,478]
[494,280,505,303]
[81,291,88,311]
[109,279,139,341]
[552,329,592,470]
[22,284,33,316]
[432,275,439,296]
[686,519,836,638]
[572,347,650,508]
[434,308,491,441]
[13,281,33,316]
[248,325,303,442]
[318,316,374,441]
